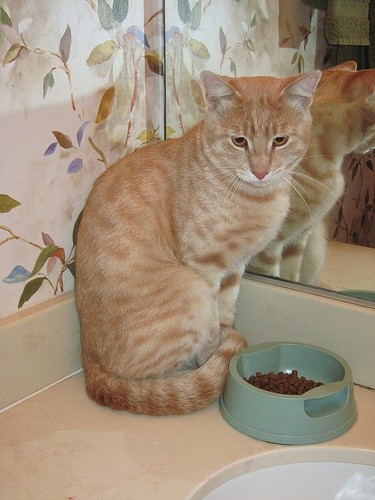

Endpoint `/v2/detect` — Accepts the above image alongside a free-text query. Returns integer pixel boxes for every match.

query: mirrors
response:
[163,0,375,309]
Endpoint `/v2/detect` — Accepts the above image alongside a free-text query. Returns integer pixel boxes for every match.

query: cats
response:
[245,61,375,286]
[74,69,323,416]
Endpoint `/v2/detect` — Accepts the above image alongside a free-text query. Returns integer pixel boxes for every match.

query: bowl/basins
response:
[219,342,357,446]
